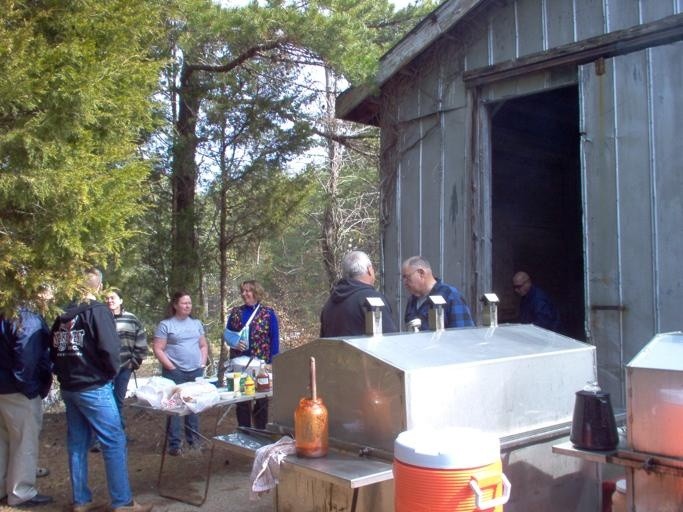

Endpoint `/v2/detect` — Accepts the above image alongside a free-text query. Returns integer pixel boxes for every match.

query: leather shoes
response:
[113,499,153,512]
[10,493,53,508]
[73,498,107,512]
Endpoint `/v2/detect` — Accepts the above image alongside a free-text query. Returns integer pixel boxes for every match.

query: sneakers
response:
[171,445,206,455]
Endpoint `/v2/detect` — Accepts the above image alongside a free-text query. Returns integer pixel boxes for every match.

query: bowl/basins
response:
[220,391,235,399]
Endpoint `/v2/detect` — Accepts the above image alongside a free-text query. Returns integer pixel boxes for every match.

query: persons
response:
[400,255,477,333]
[318,251,397,337]
[223,279,276,430]
[512,272,554,330]
[0,263,154,512]
[152,290,207,455]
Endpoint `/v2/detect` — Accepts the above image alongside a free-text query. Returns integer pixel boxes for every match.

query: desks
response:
[130,389,280,508]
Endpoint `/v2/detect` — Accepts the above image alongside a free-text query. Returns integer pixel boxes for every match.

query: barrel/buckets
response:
[392,424,512,512]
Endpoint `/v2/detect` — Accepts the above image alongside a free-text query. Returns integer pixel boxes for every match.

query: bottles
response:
[292,398,328,458]
[609,478,627,512]
[236,366,255,394]
[255,359,269,391]
